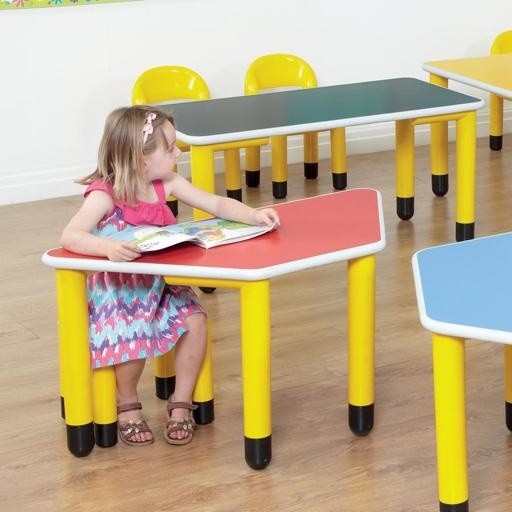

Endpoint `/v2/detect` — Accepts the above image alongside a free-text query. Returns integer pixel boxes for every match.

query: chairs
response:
[131,53,347,198]
[489,29,512,152]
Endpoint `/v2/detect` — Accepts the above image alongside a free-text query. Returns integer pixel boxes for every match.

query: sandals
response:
[117,403,154,446]
[164,393,198,445]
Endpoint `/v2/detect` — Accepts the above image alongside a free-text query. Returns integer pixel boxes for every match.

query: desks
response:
[40,186,387,471]
[412,231,511,512]
[151,77,484,294]
[423,55,512,198]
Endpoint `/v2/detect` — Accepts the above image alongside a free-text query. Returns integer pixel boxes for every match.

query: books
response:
[108,218,276,253]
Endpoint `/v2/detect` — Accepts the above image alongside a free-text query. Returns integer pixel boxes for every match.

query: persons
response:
[57,106,281,446]
